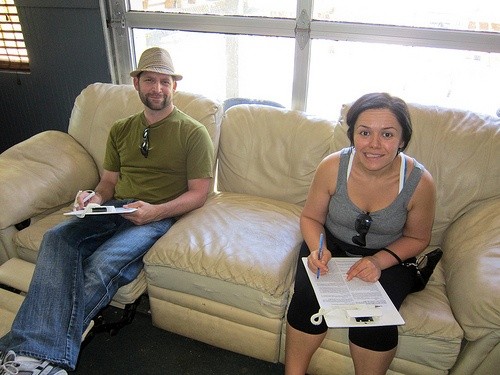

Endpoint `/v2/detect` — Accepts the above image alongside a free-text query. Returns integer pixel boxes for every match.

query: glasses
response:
[352,212,372,247]
[140,127,151,158]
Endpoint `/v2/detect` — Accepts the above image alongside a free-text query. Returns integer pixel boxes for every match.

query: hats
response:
[129,47,183,82]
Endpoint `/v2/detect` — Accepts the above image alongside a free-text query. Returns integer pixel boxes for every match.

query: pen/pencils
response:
[316,234,323,279]
[75,192,95,207]
[342,305,382,308]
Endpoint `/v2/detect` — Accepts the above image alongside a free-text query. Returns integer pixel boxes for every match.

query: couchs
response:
[0,82,500,375]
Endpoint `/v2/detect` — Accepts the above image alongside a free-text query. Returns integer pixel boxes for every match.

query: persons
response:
[0,47,214,375]
[284,93,436,375]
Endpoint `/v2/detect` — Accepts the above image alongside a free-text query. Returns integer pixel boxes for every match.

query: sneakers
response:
[0,350,69,375]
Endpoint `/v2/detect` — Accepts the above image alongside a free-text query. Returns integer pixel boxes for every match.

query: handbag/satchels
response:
[379,247,444,292]
